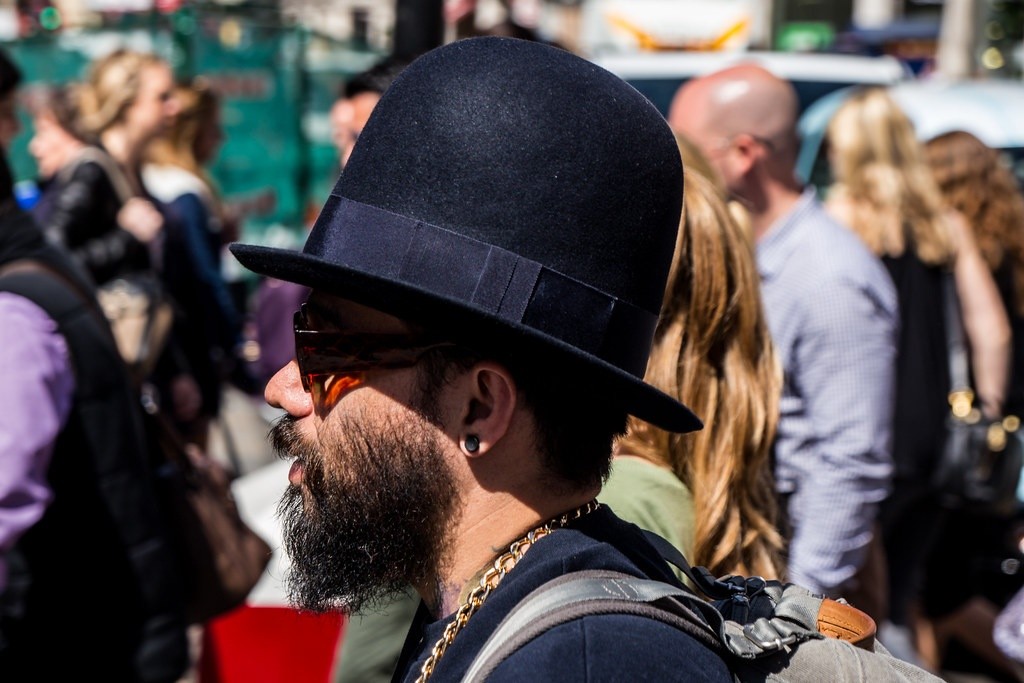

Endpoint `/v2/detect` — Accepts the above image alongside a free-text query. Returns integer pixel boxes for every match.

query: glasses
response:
[294,302,484,393]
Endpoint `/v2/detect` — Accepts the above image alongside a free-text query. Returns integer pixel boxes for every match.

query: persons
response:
[227,35,736,683]
[341,54,412,171]
[669,60,897,597]
[597,121,785,583]
[0,46,251,682]
[818,83,1010,675]
[916,131,1024,683]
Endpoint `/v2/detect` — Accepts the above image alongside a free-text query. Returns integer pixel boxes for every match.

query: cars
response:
[795,77,1024,199]
[589,52,903,121]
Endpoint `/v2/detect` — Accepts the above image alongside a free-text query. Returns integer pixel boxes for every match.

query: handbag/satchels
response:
[56,146,175,379]
[139,383,272,624]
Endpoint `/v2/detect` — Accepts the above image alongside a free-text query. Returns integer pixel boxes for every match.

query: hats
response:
[229,35,704,433]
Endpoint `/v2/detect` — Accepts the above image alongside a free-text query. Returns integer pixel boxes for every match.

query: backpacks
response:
[460,530,949,683]
[1,262,189,683]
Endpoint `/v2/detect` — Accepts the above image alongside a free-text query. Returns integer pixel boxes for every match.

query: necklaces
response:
[413,498,601,683]
